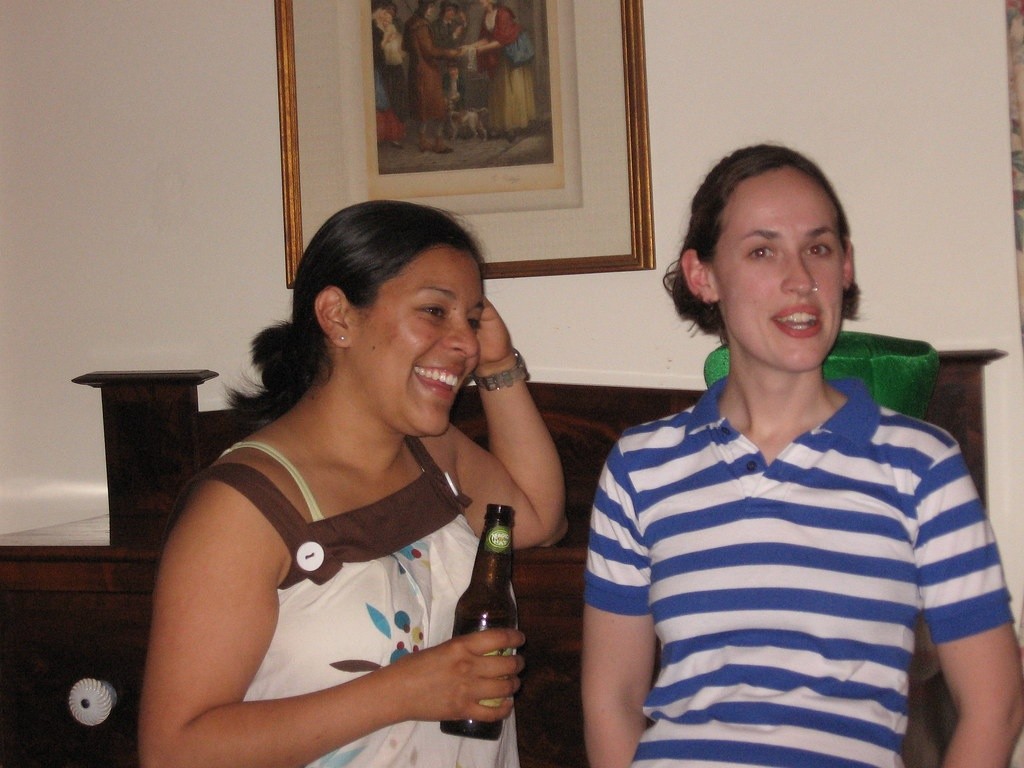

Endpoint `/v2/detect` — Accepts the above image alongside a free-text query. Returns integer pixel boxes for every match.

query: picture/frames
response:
[273,0,656,291]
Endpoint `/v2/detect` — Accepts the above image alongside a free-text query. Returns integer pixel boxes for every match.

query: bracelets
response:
[471,349,529,391]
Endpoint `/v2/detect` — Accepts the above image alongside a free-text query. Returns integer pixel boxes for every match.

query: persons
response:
[582,144,1024,768]
[137,202,568,768]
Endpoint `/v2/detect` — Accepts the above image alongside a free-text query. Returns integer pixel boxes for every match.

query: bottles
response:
[440,504,520,740]
